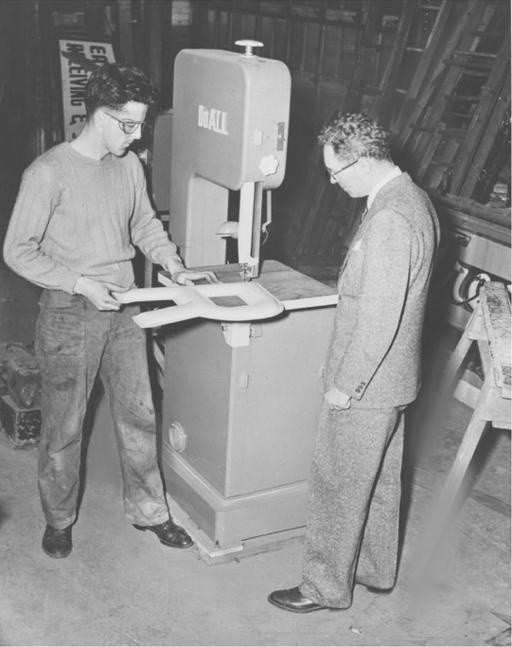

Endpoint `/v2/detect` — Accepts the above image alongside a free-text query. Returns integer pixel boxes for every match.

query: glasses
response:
[329,158,358,182]
[106,113,147,135]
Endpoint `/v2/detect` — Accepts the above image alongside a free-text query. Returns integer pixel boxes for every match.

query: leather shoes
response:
[269,586,352,613]
[42,524,71,556]
[354,577,396,594]
[134,519,194,549]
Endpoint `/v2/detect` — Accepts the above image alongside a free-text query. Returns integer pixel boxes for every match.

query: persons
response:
[2,63,222,559]
[268,109,441,613]
